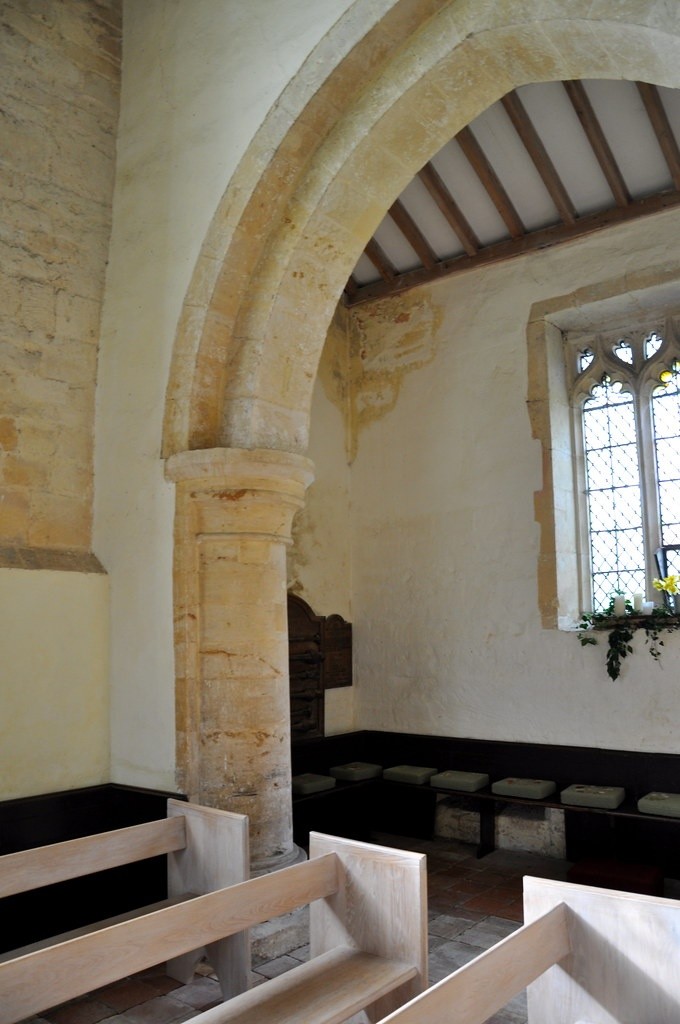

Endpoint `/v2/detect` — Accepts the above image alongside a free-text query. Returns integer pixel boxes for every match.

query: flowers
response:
[652,575,679,596]
